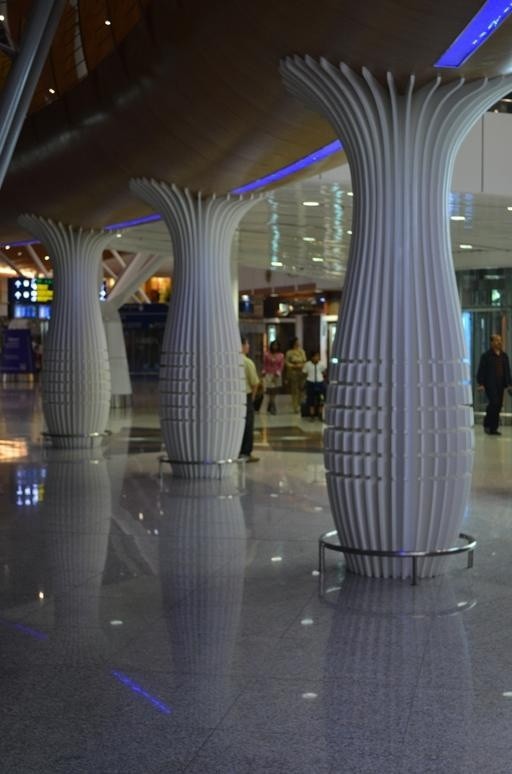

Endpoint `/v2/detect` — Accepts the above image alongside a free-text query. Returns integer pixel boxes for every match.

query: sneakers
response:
[238,453,260,463]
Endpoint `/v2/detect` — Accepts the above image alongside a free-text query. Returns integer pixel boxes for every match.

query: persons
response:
[238,334,328,462]
[477,334,512,435]
